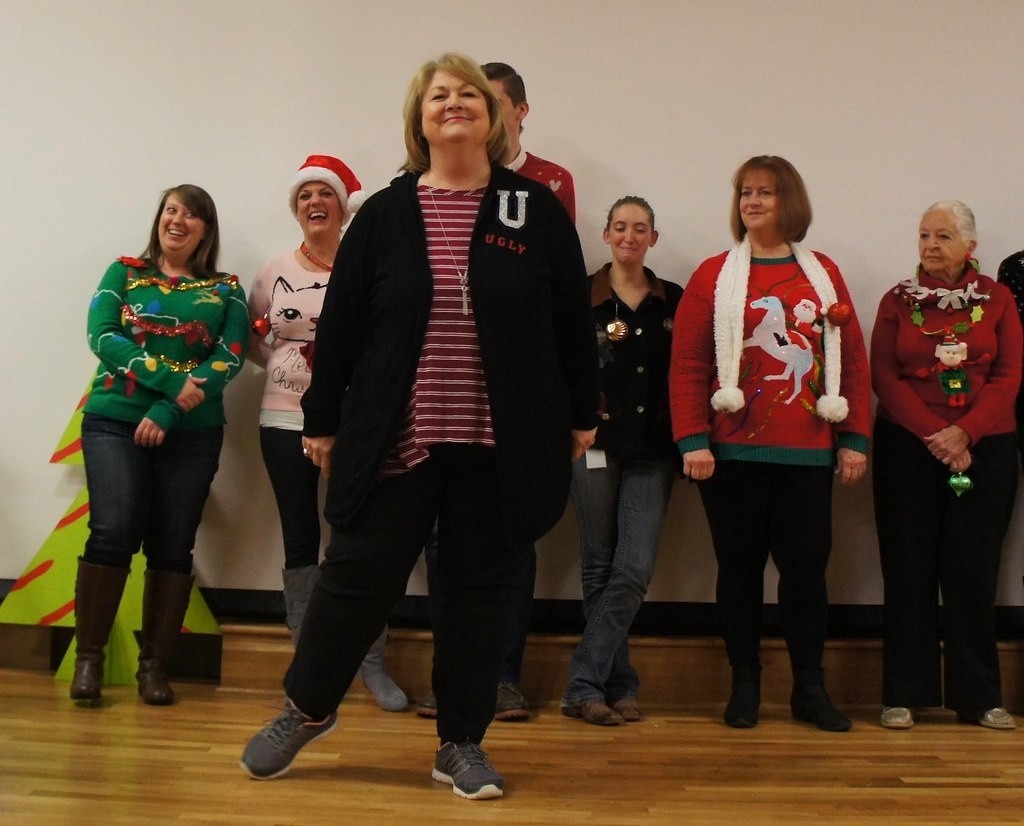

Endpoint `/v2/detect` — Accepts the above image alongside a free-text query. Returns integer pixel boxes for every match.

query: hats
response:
[289,155,367,227]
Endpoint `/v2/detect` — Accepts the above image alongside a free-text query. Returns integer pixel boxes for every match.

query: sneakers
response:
[980,706,1017,728]
[494,679,530,721]
[606,695,639,722]
[417,690,438,718]
[881,705,914,729]
[561,695,624,724]
[432,739,503,800]
[239,691,335,779]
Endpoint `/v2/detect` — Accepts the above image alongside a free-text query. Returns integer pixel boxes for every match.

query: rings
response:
[303,448,309,455]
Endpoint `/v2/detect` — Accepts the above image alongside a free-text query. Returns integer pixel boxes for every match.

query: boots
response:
[280,565,319,647]
[789,666,852,732]
[357,624,408,711]
[135,567,198,705]
[723,663,762,728]
[69,555,132,700]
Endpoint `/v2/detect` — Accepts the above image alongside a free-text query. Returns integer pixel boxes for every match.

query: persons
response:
[559,194,686,722]
[996,247,1024,471]
[238,46,600,799]
[869,196,1024,729]
[246,153,408,711]
[670,155,874,733]
[479,62,576,228]
[69,185,250,706]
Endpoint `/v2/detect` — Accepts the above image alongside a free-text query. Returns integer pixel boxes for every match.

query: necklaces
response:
[427,171,469,316]
